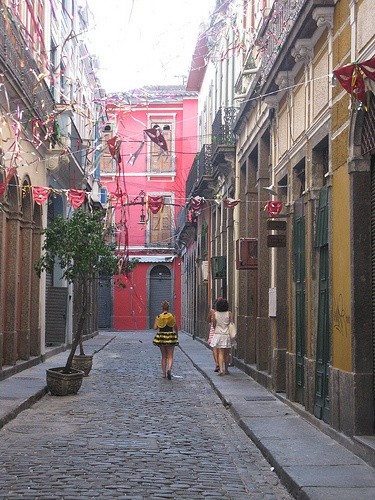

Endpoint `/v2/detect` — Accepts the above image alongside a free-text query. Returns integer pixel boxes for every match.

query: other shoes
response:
[162,374,165,378]
[167,370,171,379]
[214,364,230,375]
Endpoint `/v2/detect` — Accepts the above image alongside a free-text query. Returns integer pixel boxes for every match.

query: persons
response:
[153,301,179,380]
[209,297,235,376]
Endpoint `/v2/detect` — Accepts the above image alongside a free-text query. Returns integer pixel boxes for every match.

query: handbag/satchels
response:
[228,322,237,339]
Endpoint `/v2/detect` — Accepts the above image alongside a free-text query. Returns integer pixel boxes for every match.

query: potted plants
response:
[34,205,141,397]
